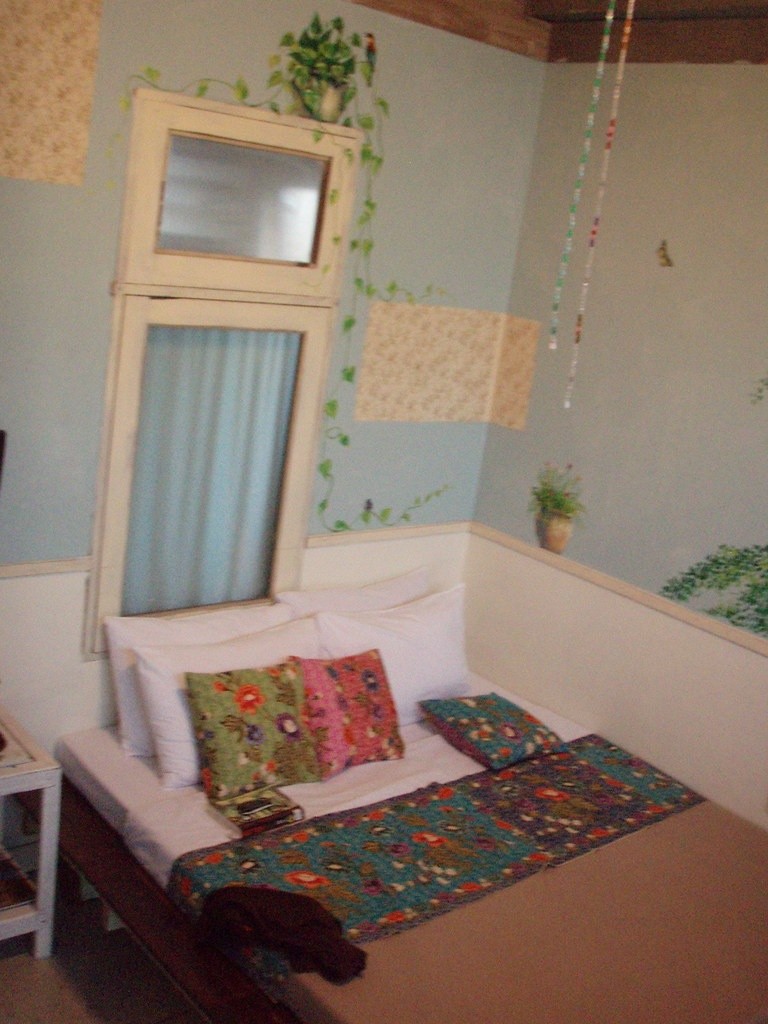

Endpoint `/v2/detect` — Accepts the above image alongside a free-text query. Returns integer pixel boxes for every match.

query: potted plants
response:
[525,459,589,556]
[103,11,455,534]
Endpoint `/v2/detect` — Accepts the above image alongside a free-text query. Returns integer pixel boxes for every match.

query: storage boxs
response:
[98,895,129,931]
[68,864,99,903]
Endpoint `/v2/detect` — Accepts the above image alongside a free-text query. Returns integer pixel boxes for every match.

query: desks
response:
[0,701,63,962]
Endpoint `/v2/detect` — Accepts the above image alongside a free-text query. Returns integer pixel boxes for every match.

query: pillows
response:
[313,584,474,728]
[183,660,323,804]
[287,648,406,784]
[271,565,431,621]
[100,602,292,759]
[128,617,320,792]
[413,690,572,772]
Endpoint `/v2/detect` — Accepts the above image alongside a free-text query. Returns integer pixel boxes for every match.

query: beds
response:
[55,665,768,1024]
